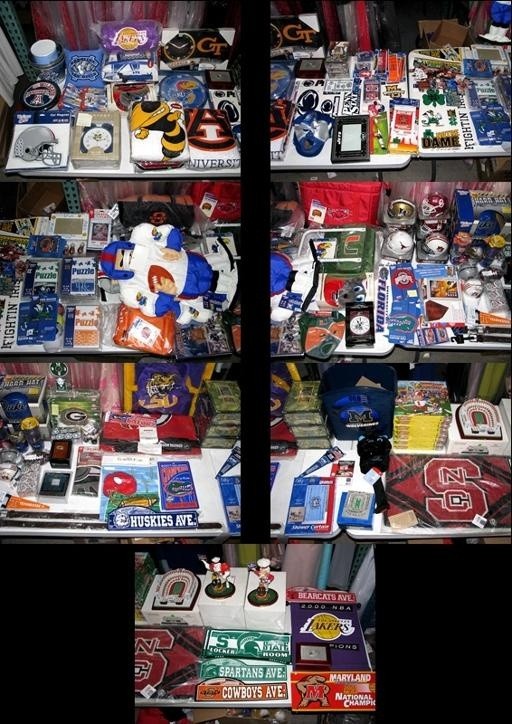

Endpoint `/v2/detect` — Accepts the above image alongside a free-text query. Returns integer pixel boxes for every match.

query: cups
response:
[26,43,66,84]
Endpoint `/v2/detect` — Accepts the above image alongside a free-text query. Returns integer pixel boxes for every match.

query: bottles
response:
[20,417,43,451]
[9,431,29,454]
[30,39,59,64]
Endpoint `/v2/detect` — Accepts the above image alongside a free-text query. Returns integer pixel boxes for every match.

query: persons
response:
[296,675,330,707]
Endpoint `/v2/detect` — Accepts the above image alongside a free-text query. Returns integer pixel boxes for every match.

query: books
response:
[70,111,122,170]
[86,209,120,251]
[3,110,72,173]
[62,305,101,350]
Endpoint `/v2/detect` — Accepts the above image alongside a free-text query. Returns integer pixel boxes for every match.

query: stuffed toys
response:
[247,558,279,608]
[201,557,236,599]
[385,189,449,258]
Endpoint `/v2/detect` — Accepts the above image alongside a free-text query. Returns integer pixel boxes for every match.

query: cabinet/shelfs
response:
[0,30,270,539]
[241,47,511,540]
